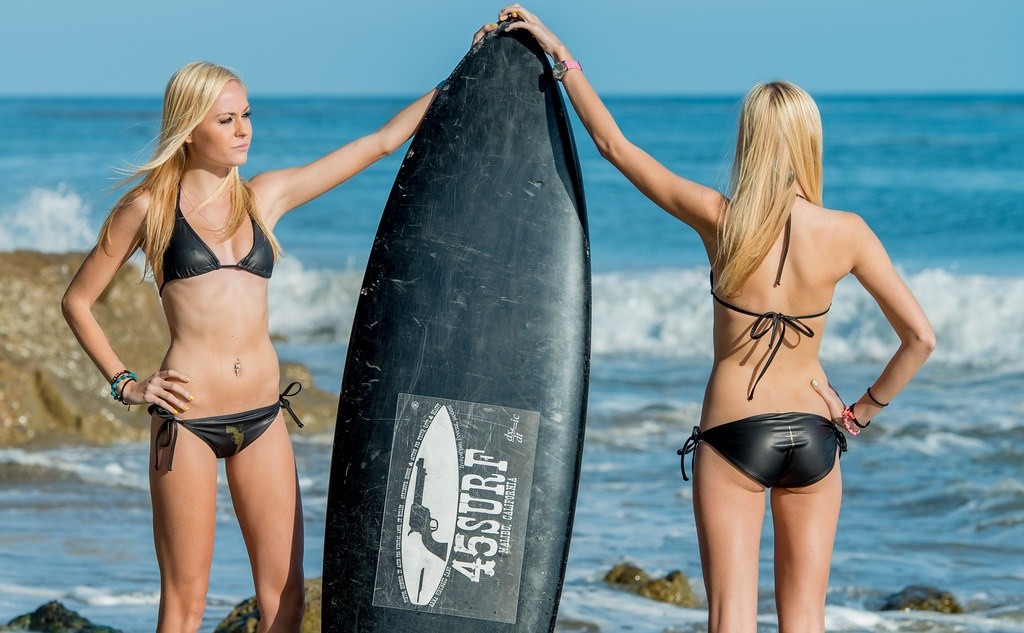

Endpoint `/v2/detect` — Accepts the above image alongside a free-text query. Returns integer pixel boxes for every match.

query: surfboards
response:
[318,15,602,633]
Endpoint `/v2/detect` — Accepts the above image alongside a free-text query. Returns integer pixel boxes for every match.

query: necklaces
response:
[178,181,226,235]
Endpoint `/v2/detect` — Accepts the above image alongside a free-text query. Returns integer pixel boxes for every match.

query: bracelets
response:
[110,370,138,412]
[866,387,889,407]
[841,403,871,436]
[435,80,447,93]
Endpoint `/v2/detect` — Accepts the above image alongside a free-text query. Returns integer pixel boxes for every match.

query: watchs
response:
[552,59,583,81]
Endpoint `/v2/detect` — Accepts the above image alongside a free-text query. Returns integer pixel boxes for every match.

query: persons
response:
[59,20,497,633]
[498,1,937,633]
